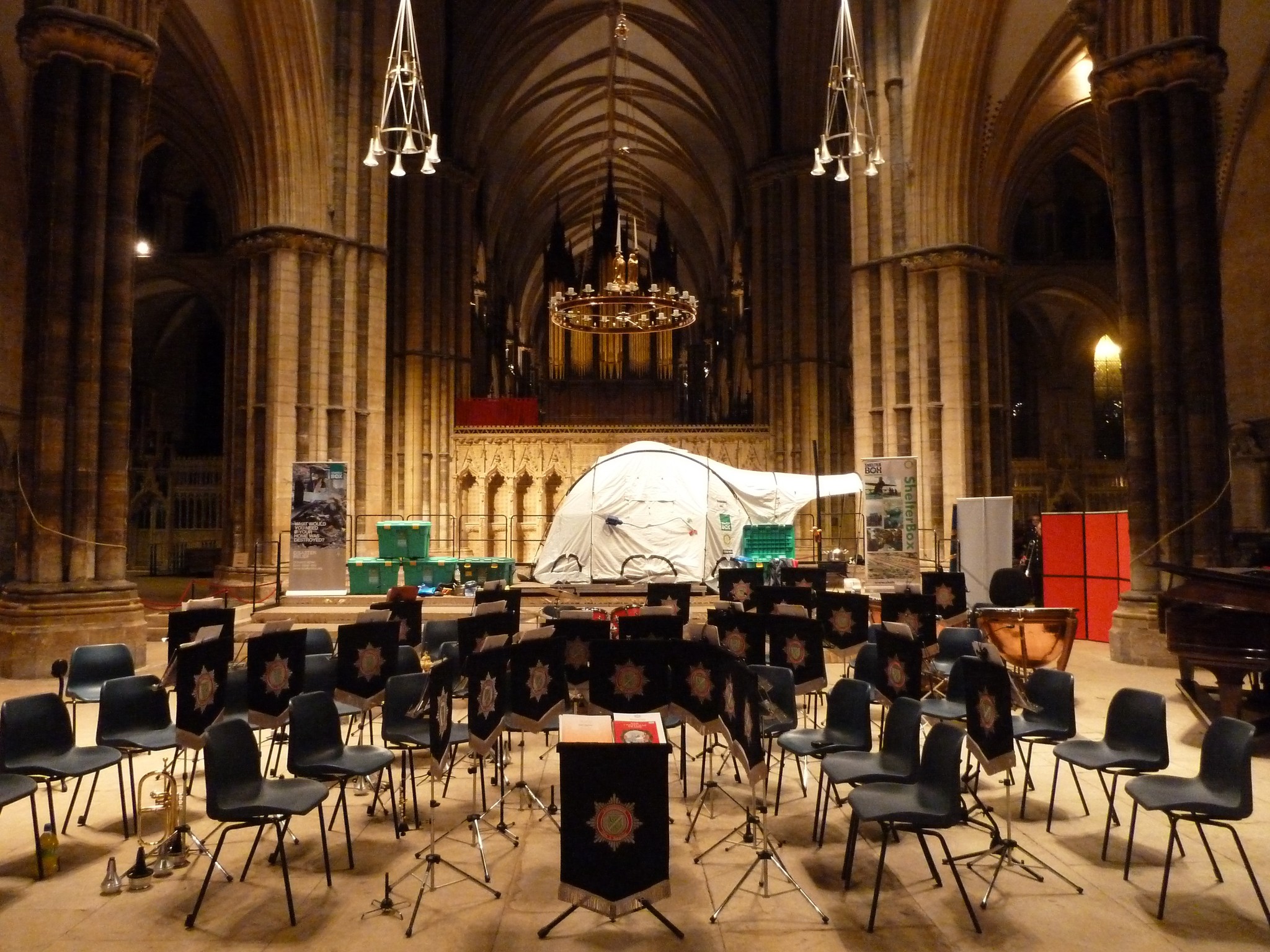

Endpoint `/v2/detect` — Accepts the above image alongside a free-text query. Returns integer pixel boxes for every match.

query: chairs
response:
[0,603,1270,933]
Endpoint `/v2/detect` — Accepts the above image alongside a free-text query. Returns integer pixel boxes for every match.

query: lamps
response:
[811,0,886,182]
[363,0,441,177]
[548,0,698,332]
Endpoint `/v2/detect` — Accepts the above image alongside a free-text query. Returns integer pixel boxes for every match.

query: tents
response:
[534,440,863,582]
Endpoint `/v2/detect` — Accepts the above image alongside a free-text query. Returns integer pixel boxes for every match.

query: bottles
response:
[126,846,154,890]
[153,843,175,877]
[101,856,122,894]
[40,823,59,878]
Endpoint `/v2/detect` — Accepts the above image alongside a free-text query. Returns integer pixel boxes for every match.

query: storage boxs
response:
[457,557,516,587]
[742,524,795,579]
[376,521,432,559]
[348,557,399,594]
[402,557,458,587]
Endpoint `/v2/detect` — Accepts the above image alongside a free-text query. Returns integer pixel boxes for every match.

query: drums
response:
[574,607,608,621]
[610,604,648,640]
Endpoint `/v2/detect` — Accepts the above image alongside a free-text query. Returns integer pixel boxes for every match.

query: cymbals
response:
[543,604,577,619]
[538,588,582,600]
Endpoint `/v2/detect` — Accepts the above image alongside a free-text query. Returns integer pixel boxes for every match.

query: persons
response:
[1020,514,1043,607]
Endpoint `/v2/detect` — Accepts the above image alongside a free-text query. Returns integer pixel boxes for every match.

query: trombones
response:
[1021,539,1035,577]
